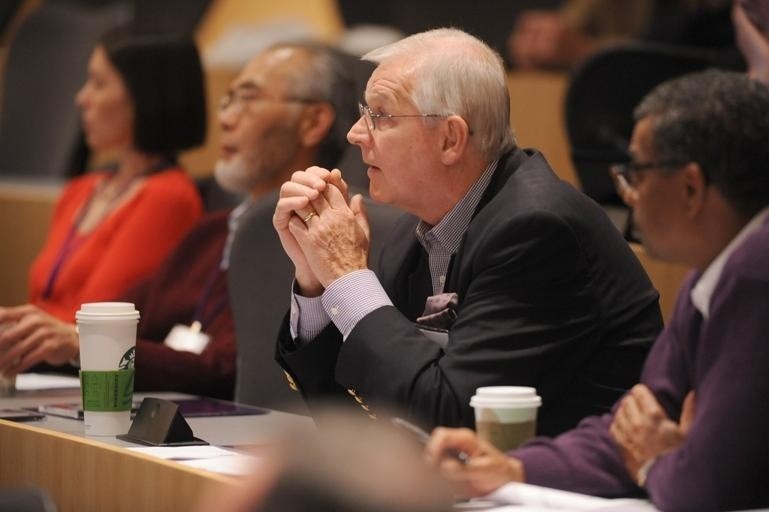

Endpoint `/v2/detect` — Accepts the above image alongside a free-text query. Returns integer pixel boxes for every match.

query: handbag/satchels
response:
[635,454,660,487]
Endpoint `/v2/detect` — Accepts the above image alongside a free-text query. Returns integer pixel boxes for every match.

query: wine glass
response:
[302,210,316,223]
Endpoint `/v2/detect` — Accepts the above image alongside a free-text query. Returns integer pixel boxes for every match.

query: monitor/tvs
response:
[471,481,660,512]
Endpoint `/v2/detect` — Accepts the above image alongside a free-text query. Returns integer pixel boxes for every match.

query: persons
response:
[0,36,359,397]
[272,22,664,454]
[29,18,208,322]
[507,0,748,241]
[420,65,768,512]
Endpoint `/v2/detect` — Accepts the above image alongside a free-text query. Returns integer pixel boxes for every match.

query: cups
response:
[359,102,474,135]
[611,161,711,194]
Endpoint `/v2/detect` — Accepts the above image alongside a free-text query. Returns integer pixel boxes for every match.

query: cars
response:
[469,386,544,454]
[74,301,141,435]
[0,320,22,395]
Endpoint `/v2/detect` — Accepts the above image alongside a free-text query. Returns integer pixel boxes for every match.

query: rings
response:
[390,417,469,463]
